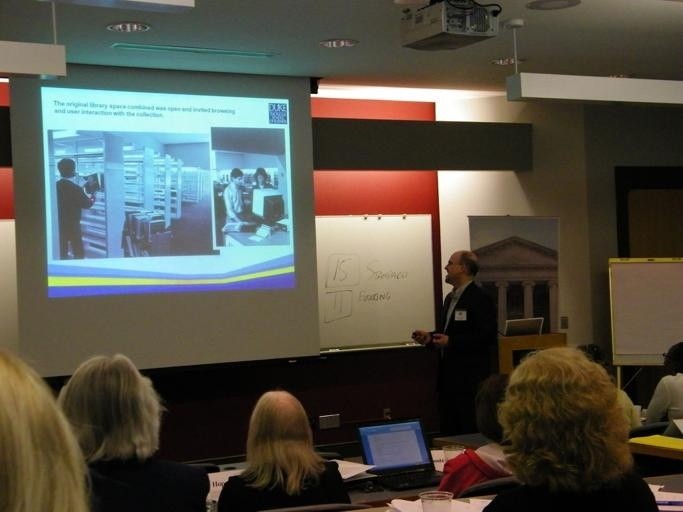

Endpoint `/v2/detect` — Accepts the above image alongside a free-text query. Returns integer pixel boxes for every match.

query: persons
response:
[411,251,499,435]
[434,374,513,498]
[577,345,643,430]
[224,168,246,224]
[56,158,96,260]
[246,167,273,213]
[56,353,210,512]
[645,342,683,423]
[218,390,351,512]
[481,346,660,512]
[0,348,91,512]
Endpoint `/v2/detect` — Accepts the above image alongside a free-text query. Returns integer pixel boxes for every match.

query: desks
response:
[193,414,682,512]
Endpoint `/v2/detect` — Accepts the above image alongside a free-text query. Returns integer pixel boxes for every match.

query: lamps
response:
[0,0,67,83]
[503,14,682,112]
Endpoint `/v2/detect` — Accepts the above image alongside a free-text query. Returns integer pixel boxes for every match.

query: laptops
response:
[354,417,451,490]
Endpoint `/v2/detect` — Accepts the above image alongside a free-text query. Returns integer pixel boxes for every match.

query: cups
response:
[418,490,454,512]
[630,406,642,425]
[442,443,466,464]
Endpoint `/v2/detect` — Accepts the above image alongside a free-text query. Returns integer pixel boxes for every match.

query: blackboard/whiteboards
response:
[0,214,435,380]
[609,257,683,366]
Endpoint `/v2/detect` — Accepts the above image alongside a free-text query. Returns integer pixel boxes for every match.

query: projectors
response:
[400,0,499,52]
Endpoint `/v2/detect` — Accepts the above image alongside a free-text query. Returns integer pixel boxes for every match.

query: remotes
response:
[412,332,427,340]
